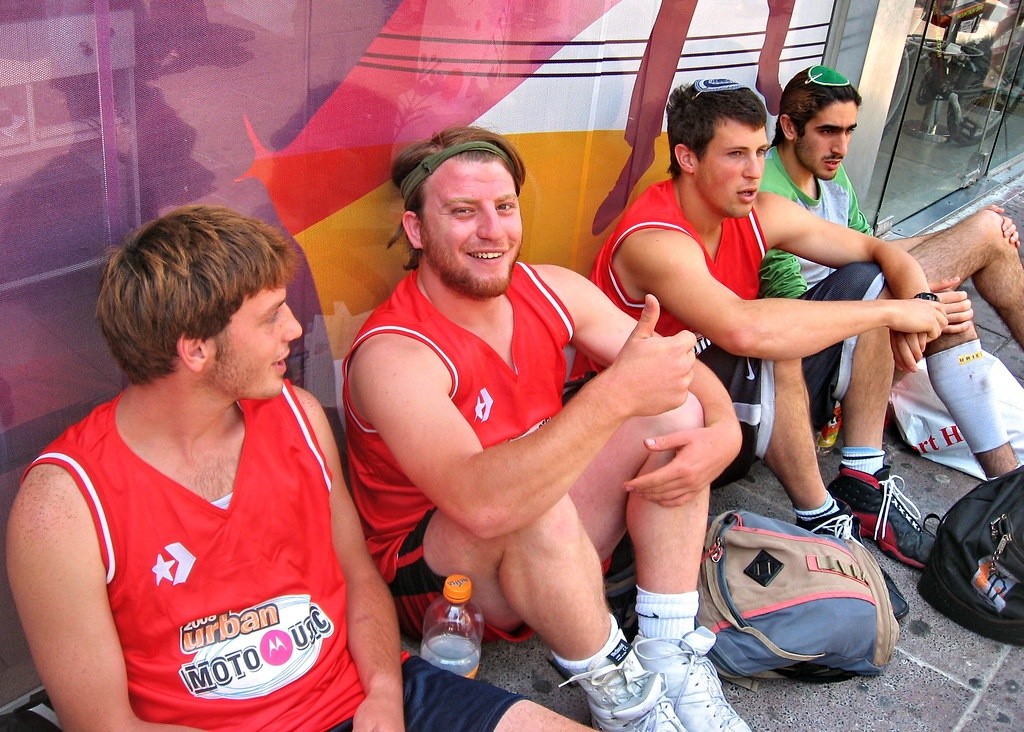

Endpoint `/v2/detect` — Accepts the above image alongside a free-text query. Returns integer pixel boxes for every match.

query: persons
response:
[758,66,1024,483]
[5,205,596,732]
[341,126,750,732]
[570,78,934,619]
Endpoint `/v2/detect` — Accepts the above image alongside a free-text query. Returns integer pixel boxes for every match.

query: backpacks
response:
[692,508,899,681]
[918,466,1024,642]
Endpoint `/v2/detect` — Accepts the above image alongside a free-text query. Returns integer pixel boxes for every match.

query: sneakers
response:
[558,629,689,732]
[796,497,909,620]
[828,464,936,569]
[631,625,751,732]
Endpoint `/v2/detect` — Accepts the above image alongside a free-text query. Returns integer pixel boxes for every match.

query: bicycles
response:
[882,13,1024,147]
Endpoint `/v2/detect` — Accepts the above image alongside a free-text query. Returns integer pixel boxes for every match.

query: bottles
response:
[418,573,480,679]
[814,400,842,453]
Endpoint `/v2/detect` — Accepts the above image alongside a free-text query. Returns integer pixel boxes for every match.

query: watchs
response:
[910,292,941,302]
